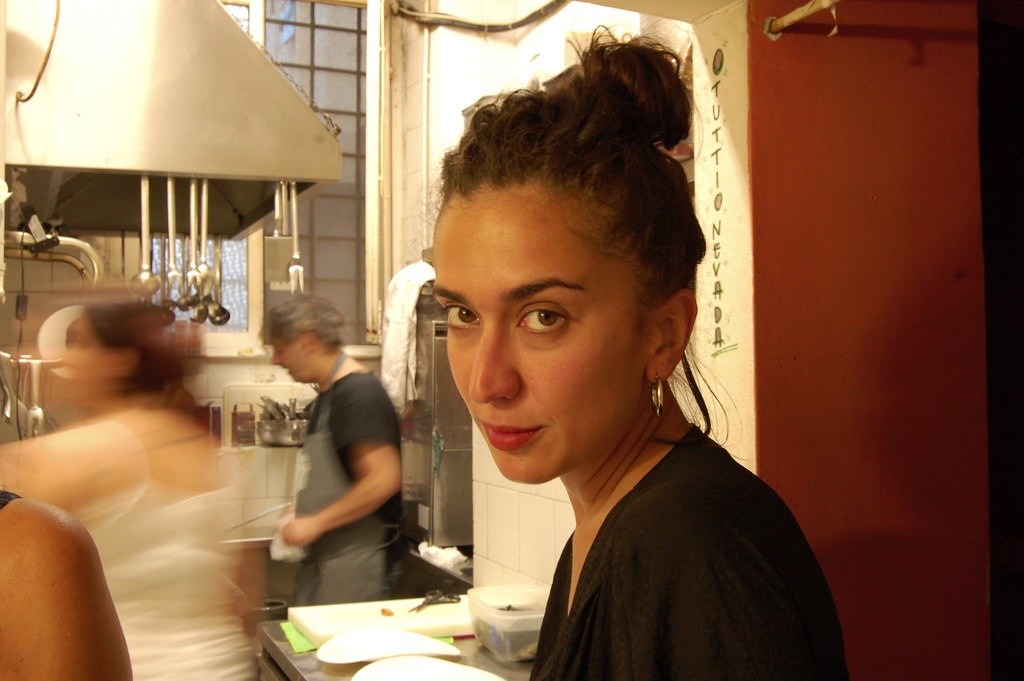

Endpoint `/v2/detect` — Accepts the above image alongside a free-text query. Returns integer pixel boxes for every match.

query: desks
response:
[256,620,534,681]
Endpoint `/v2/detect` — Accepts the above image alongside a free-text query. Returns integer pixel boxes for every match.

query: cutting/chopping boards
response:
[288,594,472,652]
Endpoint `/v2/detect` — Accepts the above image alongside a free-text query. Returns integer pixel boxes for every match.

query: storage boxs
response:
[468,584,550,663]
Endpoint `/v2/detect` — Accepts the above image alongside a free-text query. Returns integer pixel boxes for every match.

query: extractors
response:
[0,0,341,239]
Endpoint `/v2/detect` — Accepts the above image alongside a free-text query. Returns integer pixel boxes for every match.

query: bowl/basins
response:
[467,584,549,661]
[258,420,309,446]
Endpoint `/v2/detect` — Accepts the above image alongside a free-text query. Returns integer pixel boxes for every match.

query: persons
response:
[1,288,407,681]
[431,33,849,681]
